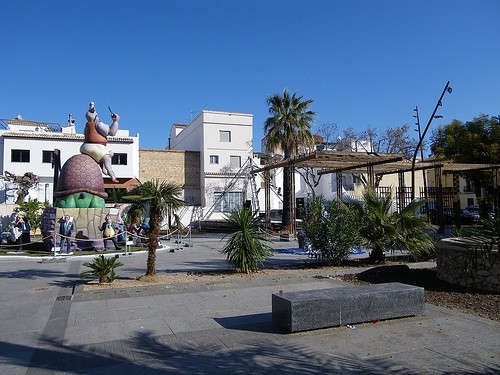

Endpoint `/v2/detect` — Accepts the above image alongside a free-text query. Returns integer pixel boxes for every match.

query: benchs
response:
[272,282,426,331]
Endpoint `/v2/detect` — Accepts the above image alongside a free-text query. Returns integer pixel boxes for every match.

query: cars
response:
[488,210,496,220]
[426,206,479,224]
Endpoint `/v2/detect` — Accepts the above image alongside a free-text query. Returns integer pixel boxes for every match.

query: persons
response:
[321,207,328,219]
[14,215,25,252]
[98,216,122,251]
[56,215,74,255]
[131,218,140,246]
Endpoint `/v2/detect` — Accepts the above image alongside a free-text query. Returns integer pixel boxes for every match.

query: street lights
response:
[44,183,50,203]
